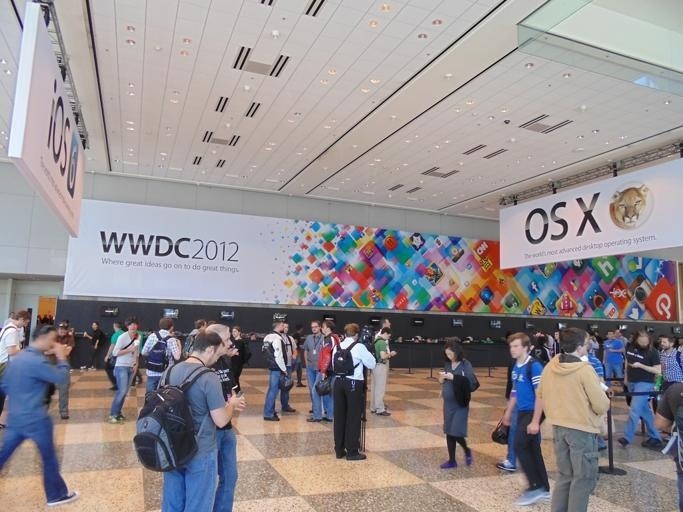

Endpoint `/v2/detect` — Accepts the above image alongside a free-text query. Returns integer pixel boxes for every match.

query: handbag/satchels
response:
[462,358,480,392]
[109,356,116,366]
[492,419,509,444]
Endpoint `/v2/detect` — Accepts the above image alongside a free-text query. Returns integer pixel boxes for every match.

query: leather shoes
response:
[138,377,142,384]
[371,407,387,413]
[131,381,135,386]
[641,437,661,447]
[109,384,118,390]
[618,437,629,448]
[263,404,332,423]
[376,412,391,416]
[336,450,346,458]
[0,424,5,429]
[296,382,306,387]
[346,453,366,460]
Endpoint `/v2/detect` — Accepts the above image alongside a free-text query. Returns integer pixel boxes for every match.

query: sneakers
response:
[88,367,96,370]
[515,484,545,505]
[116,414,125,420]
[440,461,457,469]
[61,411,69,419]
[466,448,472,465]
[81,366,86,368]
[46,490,79,507]
[107,415,119,423]
[540,490,551,499]
[495,459,517,472]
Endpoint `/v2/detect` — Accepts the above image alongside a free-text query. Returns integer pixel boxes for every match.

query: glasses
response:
[57,323,69,327]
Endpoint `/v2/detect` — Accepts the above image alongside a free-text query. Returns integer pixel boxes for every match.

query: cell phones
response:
[234,390,244,399]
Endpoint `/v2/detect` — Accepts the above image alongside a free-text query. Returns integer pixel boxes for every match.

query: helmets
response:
[279,374,294,392]
[316,379,331,396]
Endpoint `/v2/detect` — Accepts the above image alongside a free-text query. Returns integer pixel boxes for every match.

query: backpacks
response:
[135,364,212,471]
[363,339,382,361]
[334,341,361,375]
[145,333,172,372]
